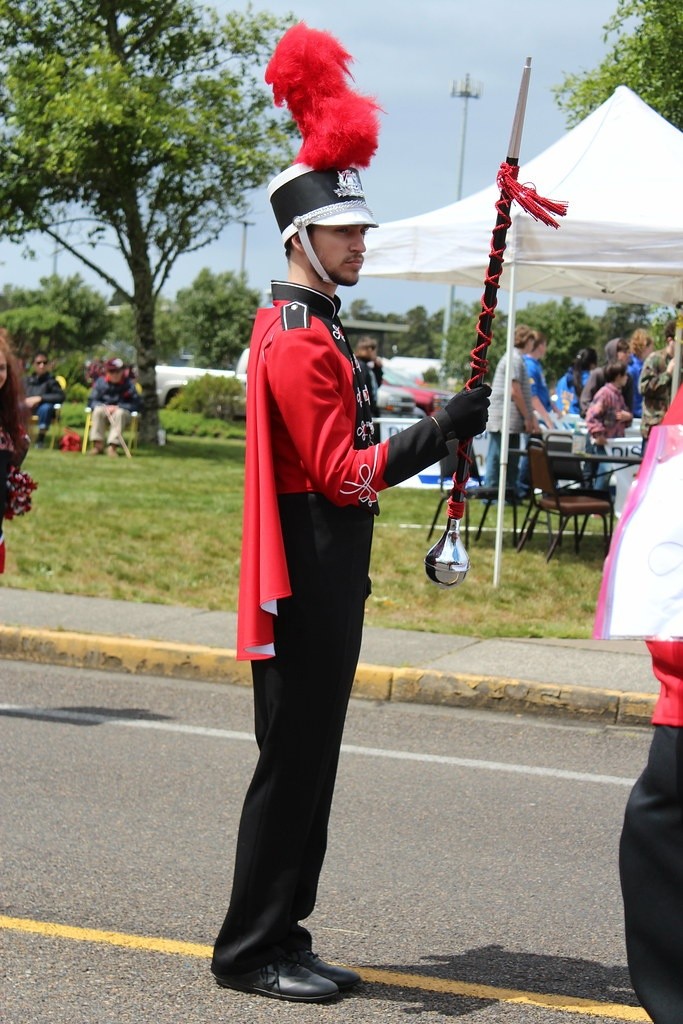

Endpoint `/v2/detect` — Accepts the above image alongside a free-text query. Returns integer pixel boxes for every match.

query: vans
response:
[388,357,464,386]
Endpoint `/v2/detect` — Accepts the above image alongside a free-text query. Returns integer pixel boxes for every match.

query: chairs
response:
[520,434,613,542]
[516,435,613,563]
[30,374,67,450]
[426,439,519,552]
[82,383,143,455]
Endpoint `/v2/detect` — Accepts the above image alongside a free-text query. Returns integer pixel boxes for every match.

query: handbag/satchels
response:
[59,427,81,452]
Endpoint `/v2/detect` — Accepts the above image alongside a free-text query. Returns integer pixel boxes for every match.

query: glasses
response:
[106,370,122,374]
[33,361,47,365]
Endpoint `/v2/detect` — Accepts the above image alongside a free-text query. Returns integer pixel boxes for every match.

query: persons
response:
[85,358,142,456]
[355,334,383,444]
[617,382,683,1024]
[0,329,30,576]
[21,353,66,449]
[213,160,491,1001]
[481,321,683,507]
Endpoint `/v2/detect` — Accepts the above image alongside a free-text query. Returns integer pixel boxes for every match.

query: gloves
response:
[431,383,493,441]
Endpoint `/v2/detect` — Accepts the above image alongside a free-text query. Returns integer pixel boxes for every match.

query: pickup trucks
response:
[155,347,416,418]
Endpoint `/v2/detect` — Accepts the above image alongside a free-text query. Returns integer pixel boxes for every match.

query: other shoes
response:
[87,446,119,458]
[34,442,42,450]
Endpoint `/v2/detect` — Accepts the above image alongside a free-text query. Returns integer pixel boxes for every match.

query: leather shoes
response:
[211,948,360,1002]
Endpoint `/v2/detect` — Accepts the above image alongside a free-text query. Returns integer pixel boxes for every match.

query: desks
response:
[507,446,643,557]
[372,417,491,489]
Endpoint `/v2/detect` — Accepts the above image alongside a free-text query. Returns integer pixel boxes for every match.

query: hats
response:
[105,358,123,369]
[267,161,379,250]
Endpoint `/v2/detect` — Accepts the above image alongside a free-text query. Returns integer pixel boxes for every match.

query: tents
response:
[359,85,683,588]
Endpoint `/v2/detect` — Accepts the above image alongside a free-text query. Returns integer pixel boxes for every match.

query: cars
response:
[414,378,458,411]
[382,369,435,416]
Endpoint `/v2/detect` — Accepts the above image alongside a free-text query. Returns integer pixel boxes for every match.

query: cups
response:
[158,430,166,446]
